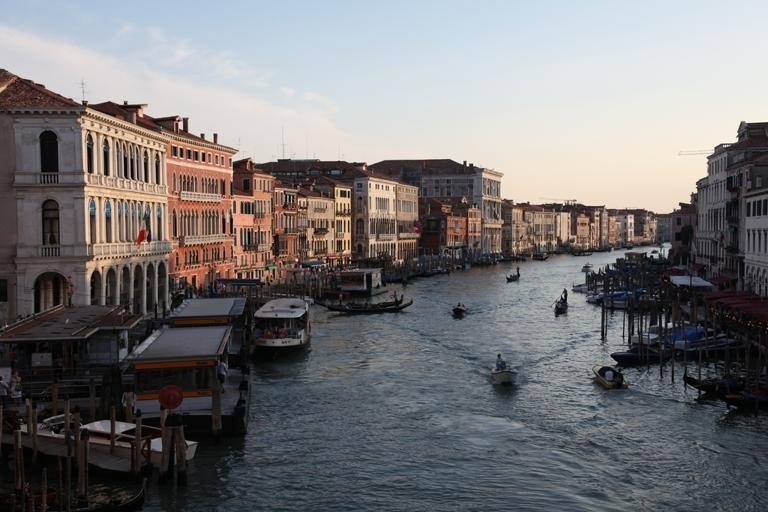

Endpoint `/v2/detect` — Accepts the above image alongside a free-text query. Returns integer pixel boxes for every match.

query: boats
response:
[506,273,520,281]
[314,295,413,314]
[554,297,568,314]
[582,263,593,272]
[492,367,518,386]
[574,281,661,308]
[592,364,628,389]
[684,374,767,409]
[634,319,746,355]
[452,302,466,314]
[254,297,313,349]
[1,411,200,469]
[610,345,669,364]
[120,296,254,435]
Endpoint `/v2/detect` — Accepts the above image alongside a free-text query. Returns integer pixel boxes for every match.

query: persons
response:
[254,324,289,338]
[185,281,213,299]
[0,376,9,409]
[605,367,614,381]
[9,369,23,408]
[563,287,568,302]
[339,293,343,305]
[496,353,506,370]
[516,267,519,275]
[458,302,460,307]
[218,355,228,394]
